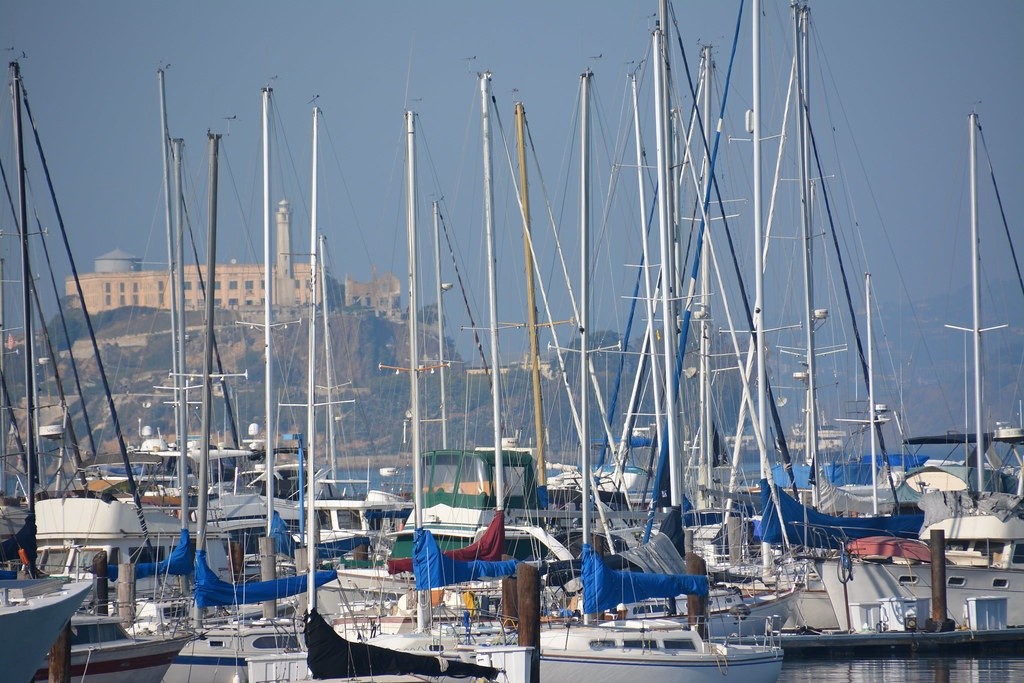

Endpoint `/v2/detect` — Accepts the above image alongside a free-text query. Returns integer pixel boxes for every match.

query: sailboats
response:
[2,3,1024,682]
